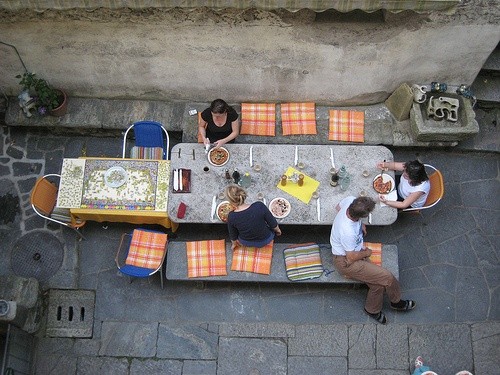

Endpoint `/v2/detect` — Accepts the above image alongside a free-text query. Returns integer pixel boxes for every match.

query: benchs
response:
[182,102,394,146]
[166,240,400,286]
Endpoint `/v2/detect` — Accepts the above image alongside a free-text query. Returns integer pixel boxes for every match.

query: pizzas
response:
[210,148,227,165]
[218,202,235,222]
[373,177,391,194]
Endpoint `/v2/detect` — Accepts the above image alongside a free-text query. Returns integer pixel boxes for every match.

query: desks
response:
[56,156,179,232]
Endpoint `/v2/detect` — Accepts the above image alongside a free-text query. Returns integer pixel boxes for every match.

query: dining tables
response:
[167,142,398,227]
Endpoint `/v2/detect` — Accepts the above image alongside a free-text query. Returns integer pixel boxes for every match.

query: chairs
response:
[31,173,89,241]
[116,228,169,291]
[397,163,445,224]
[123,120,170,162]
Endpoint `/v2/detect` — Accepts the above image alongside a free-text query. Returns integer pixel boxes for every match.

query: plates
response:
[374,173,395,193]
[216,200,230,220]
[269,198,291,219]
[104,166,127,188]
[208,146,230,167]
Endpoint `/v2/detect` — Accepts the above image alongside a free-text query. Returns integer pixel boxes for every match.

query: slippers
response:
[363,308,387,325]
[390,300,416,311]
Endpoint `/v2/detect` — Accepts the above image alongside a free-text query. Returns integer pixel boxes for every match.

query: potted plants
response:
[15,68,67,118]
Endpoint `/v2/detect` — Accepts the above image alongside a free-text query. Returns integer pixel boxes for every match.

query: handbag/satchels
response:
[177,200,190,219]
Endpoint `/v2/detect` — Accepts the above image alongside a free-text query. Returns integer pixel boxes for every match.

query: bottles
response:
[242,172,251,188]
[341,175,351,191]
[225,168,230,179]
[232,168,240,184]
[330,172,338,187]
[338,165,346,180]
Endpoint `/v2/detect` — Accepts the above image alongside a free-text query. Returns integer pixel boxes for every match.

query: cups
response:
[362,169,370,177]
[203,166,210,173]
[257,192,264,200]
[329,168,337,174]
[298,162,305,169]
[360,190,367,197]
[312,191,320,198]
[254,164,261,171]
[218,192,225,200]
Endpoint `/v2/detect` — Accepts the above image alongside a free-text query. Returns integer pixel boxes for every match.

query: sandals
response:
[415,356,424,367]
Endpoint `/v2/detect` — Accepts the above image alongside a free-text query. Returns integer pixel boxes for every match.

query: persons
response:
[377,159,431,212]
[224,184,282,251]
[198,98,240,150]
[330,196,416,325]
